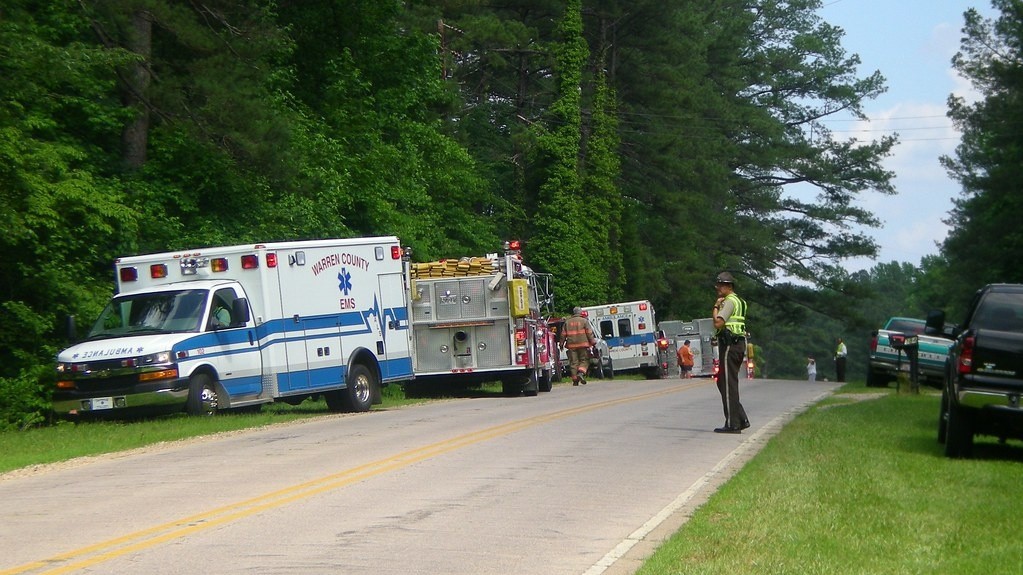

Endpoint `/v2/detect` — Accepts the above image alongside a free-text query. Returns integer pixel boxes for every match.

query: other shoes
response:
[578,372,587,384]
[573,380,578,387]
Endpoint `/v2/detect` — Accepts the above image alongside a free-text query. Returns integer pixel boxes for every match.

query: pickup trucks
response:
[867,317,957,390]
[938,283,1023,457]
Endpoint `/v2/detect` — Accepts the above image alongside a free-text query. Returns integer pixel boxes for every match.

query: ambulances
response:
[580,299,668,379]
[51,234,418,420]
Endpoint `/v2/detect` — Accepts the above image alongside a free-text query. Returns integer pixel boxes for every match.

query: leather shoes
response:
[714,418,751,434]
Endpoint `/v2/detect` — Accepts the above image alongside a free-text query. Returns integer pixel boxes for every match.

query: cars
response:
[548,313,615,380]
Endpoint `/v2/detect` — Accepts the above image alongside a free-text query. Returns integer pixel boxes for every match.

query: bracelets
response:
[713,306,719,310]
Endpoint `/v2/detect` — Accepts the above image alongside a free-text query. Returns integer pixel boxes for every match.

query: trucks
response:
[657,317,755,379]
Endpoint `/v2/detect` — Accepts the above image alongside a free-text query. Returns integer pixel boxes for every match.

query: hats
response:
[713,272,737,284]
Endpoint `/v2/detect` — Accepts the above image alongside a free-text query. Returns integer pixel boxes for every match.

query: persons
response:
[806,355,816,382]
[201,294,231,327]
[834,337,847,382]
[560,307,597,386]
[677,340,694,379]
[713,272,750,434]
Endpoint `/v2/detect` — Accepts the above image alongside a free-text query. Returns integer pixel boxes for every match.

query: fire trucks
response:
[412,241,565,397]
